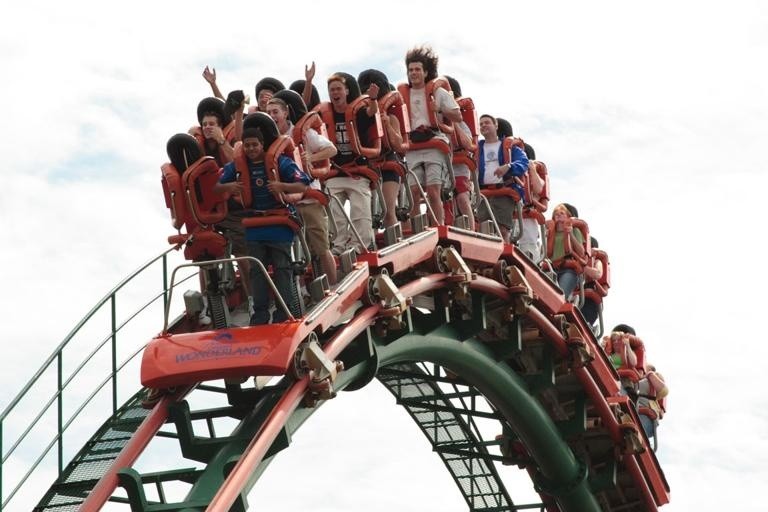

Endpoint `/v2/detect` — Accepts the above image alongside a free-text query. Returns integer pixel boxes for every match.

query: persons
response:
[604,331,668,437]
[190,61,337,325]
[552,204,610,325]
[405,48,476,231]
[324,74,403,254]
[478,115,548,264]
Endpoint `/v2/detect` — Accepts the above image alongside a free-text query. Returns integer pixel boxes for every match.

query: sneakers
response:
[198,307,211,324]
[248,303,254,318]
[332,245,361,255]
